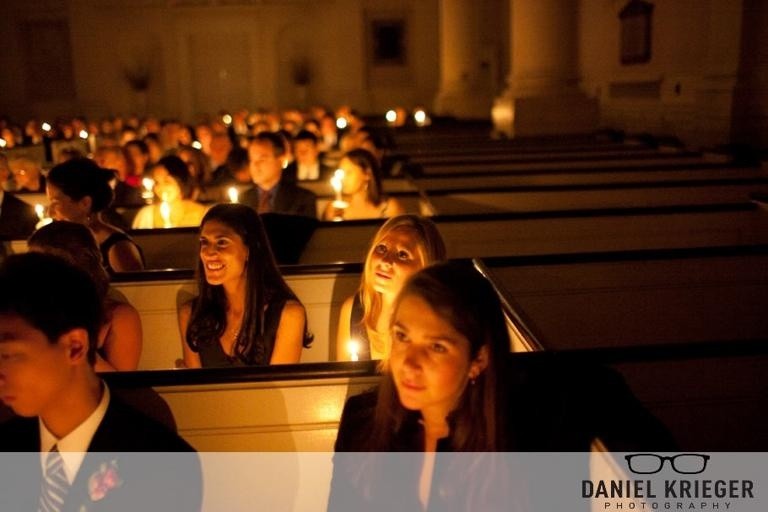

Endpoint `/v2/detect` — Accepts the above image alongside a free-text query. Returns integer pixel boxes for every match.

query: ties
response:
[257,191,274,214]
[37,445,70,512]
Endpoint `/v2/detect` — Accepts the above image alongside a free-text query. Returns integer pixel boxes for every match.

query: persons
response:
[1,106,432,272]
[176,202,314,370]
[0,251,204,512]
[337,213,447,361]
[328,264,590,512]
[25,221,143,374]
